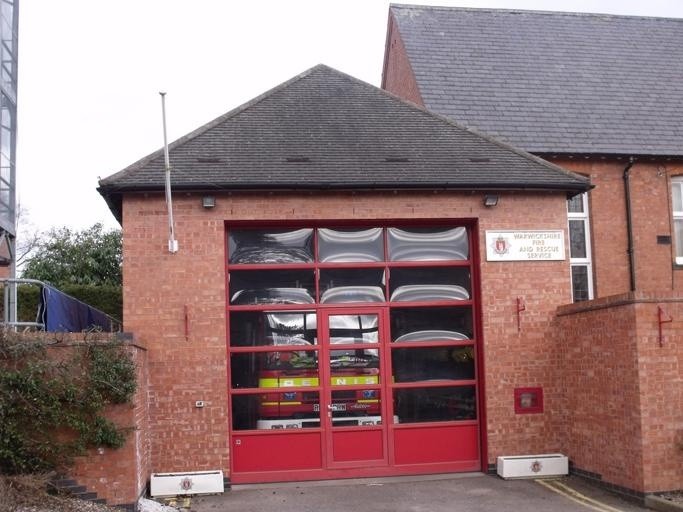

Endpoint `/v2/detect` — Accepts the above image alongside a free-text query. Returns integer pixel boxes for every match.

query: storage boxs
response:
[150,470,224,499]
[497,453,568,481]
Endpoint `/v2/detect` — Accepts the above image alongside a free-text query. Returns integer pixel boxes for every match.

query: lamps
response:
[202,193,215,207]
[484,195,498,206]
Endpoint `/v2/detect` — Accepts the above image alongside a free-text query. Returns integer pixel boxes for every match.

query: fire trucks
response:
[232,284,402,430]
